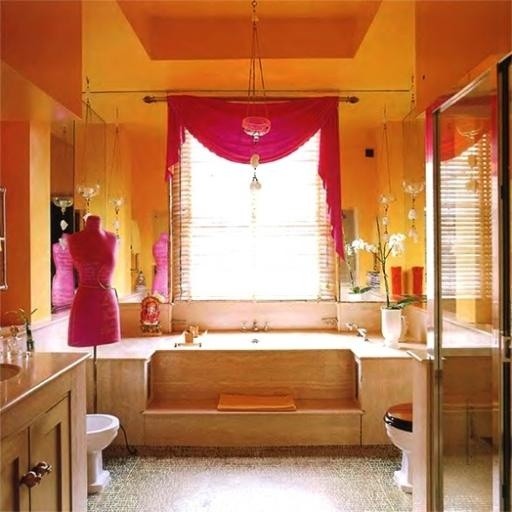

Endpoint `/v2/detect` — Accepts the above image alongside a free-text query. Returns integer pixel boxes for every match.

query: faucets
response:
[252,320,259,332]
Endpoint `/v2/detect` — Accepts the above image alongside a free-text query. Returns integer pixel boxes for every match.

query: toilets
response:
[383,404,412,493]
[85,413,120,495]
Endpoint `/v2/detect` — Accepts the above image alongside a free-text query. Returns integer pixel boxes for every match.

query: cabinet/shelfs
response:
[1,359,88,512]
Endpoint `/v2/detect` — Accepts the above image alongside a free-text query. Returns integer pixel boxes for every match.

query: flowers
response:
[344,243,372,294]
[351,233,419,308]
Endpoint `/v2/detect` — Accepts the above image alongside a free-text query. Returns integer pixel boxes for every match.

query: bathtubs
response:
[161,333,357,350]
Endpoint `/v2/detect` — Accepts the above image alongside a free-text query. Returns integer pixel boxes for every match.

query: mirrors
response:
[111,91,170,305]
[342,104,426,302]
[51,105,79,313]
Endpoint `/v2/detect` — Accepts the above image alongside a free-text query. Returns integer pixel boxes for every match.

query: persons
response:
[151,231,170,304]
[52,233,75,310]
[63,215,122,348]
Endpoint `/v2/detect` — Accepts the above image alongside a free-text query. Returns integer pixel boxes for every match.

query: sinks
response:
[0,363,20,381]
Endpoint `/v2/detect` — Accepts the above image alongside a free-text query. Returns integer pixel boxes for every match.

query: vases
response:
[351,293,364,301]
[380,309,404,348]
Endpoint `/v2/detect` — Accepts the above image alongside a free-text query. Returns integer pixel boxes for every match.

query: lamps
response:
[241,2,270,194]
[78,78,101,223]
[109,107,127,231]
[402,74,425,233]
[377,104,396,235]
[52,196,74,230]
